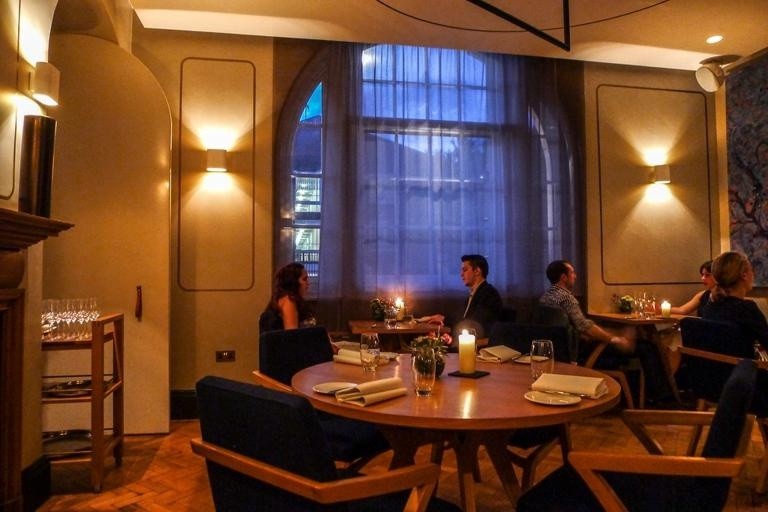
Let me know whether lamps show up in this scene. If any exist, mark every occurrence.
[693,63,746,94]
[24,61,62,108]
[647,164,671,186]
[203,148,233,174]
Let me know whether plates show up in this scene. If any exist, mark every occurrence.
[311,382,357,396]
[523,390,582,407]
[513,355,548,364]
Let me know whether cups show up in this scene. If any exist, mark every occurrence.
[632,293,655,320]
[407,307,413,324]
[360,332,380,373]
[530,341,555,379]
[367,309,376,328]
[410,347,436,396]
[383,308,396,330]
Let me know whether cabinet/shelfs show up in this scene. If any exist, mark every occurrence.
[39,309,127,494]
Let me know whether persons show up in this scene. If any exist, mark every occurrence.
[665,261,720,372]
[539,261,659,389]
[705,252,768,364]
[259,263,310,330]
[423,255,504,337]
